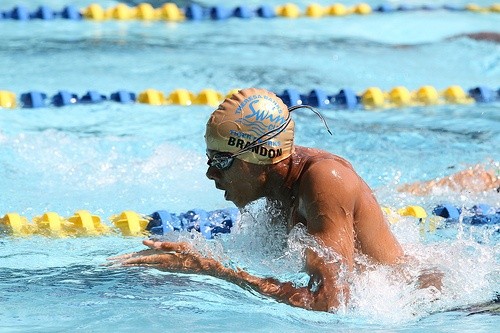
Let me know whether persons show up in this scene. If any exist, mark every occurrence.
[204,88,404,263]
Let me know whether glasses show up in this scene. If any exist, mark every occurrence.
[205,104,332,171]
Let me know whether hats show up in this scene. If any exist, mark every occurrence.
[205,87,295,164]
